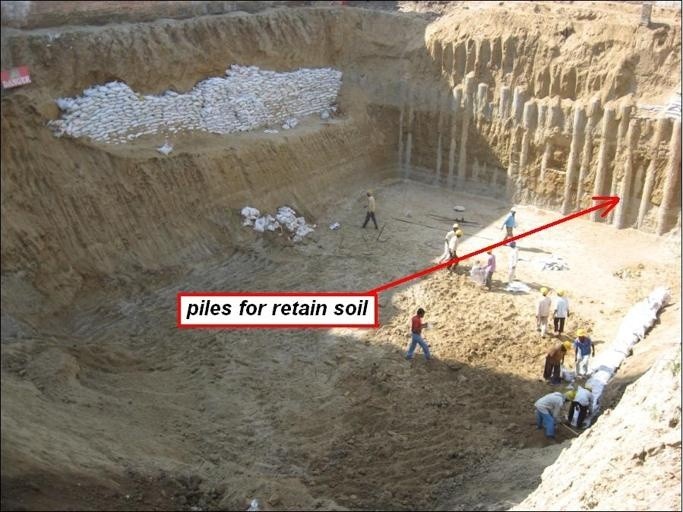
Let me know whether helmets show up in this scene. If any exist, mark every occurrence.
[563,341,572,352]
[511,206,517,212]
[584,385,592,391]
[539,287,549,295]
[575,329,586,337]
[453,223,463,236]
[565,390,576,400]
[557,288,564,295]
[486,248,492,253]
[366,189,374,195]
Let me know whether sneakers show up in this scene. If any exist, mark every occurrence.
[534,327,562,338]
[535,421,586,439]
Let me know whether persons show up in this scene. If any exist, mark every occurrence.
[572,328,595,380]
[534,287,552,338]
[498,207,518,248]
[476,248,496,291]
[532,390,574,440]
[549,288,570,336]
[542,340,571,386]
[436,223,458,264]
[445,230,463,271]
[504,240,517,284]
[469,259,482,298]
[403,308,435,361]
[360,189,378,230]
[563,384,594,430]
